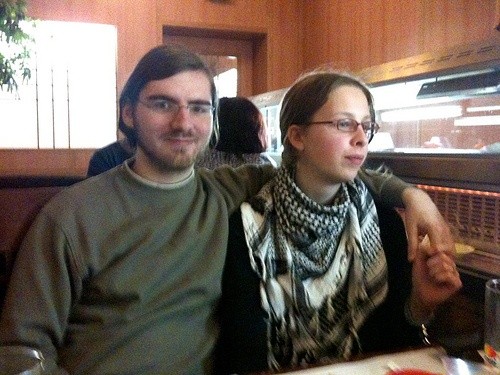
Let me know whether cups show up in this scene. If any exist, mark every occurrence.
[0,346,45,375]
[484,279,500,367]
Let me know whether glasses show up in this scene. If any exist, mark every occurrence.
[137,99,215,113]
[287,118,379,134]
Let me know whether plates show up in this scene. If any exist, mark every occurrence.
[454,243,475,258]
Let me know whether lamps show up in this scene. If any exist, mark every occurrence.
[416,70,500,99]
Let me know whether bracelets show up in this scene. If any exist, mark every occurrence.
[403,299,436,328]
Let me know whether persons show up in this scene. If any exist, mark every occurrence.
[223,67,463,375]
[194,96,276,167]
[86,125,134,178]
[0,43,459,375]
